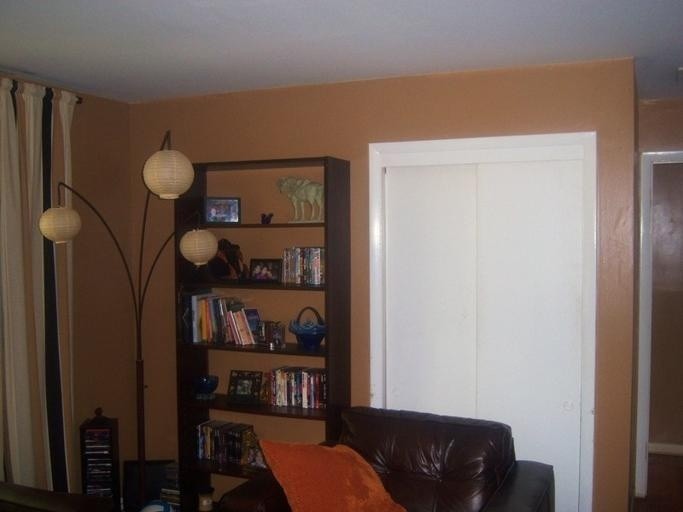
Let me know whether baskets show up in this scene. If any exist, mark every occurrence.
[289,307,325,347]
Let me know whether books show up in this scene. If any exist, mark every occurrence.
[269,366,327,410]
[180,289,259,346]
[281,245,324,285]
[192,419,254,465]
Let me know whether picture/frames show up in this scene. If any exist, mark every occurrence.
[228,369,263,400]
[203,195,242,224]
[249,258,283,283]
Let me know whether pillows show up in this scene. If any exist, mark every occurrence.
[257,440,406,512]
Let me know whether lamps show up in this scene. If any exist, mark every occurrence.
[40,129,218,459]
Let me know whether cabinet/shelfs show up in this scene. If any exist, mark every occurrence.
[173,155,352,484]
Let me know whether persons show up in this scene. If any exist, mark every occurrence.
[256,264,272,280]
[252,263,261,277]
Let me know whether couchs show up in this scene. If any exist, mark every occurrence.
[214,403,555,512]
